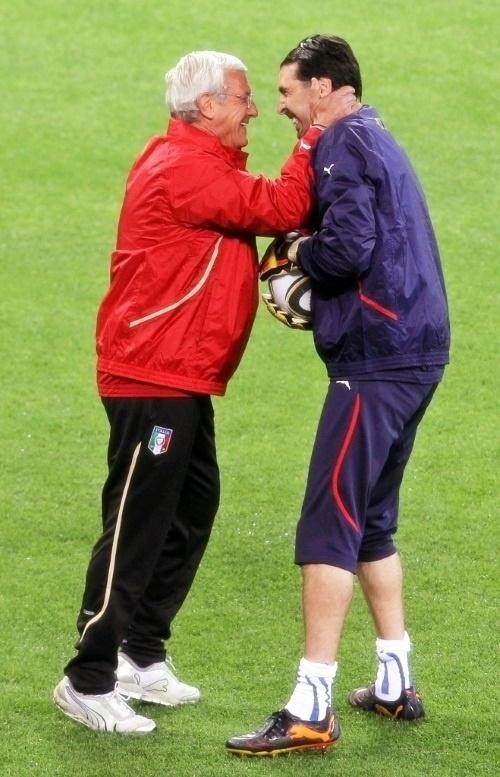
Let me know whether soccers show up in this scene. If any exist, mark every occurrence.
[268,265,314,320]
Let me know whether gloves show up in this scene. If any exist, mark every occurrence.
[257,229,312,281]
[262,292,316,331]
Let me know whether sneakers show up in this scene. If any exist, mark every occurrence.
[116,652,200,707]
[52,675,157,734]
[349,686,424,721]
[226,709,342,758]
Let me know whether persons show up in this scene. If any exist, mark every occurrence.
[222,29,456,756]
[48,48,364,739]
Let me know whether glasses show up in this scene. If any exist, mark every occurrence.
[217,91,255,109]
